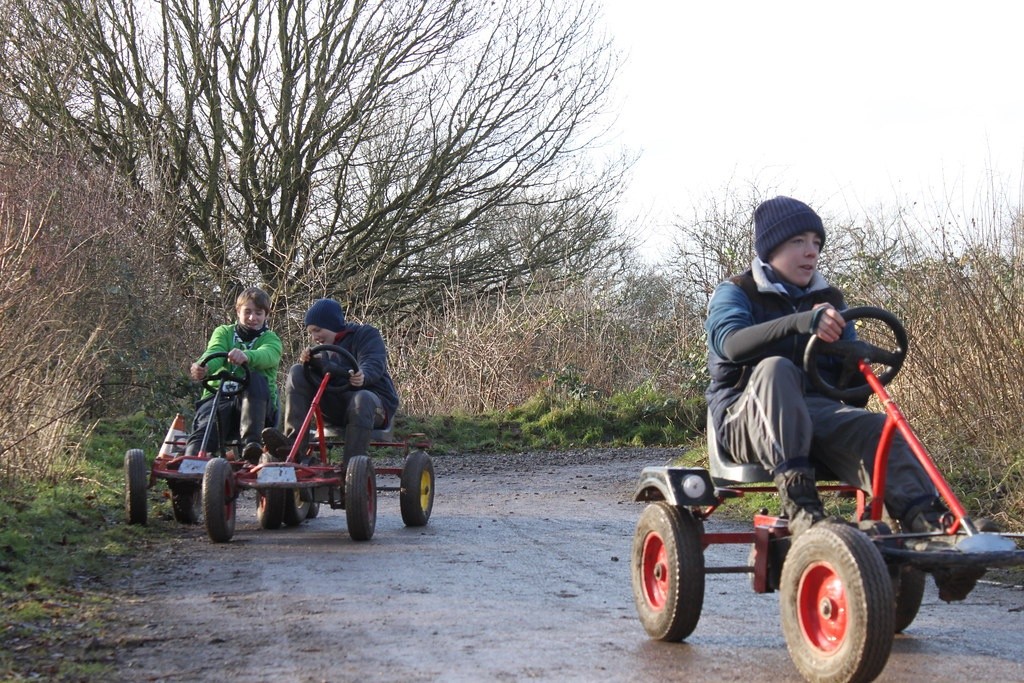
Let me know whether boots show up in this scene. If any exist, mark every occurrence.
[185,441,201,457]
[260,394,309,463]
[907,508,1004,603]
[772,456,848,549]
[335,426,372,485]
[240,396,263,465]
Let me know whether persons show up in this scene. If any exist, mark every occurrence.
[261,300,399,479]
[705,196,999,602]
[185,287,282,459]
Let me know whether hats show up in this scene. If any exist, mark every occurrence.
[304,299,345,334]
[754,196,826,263]
